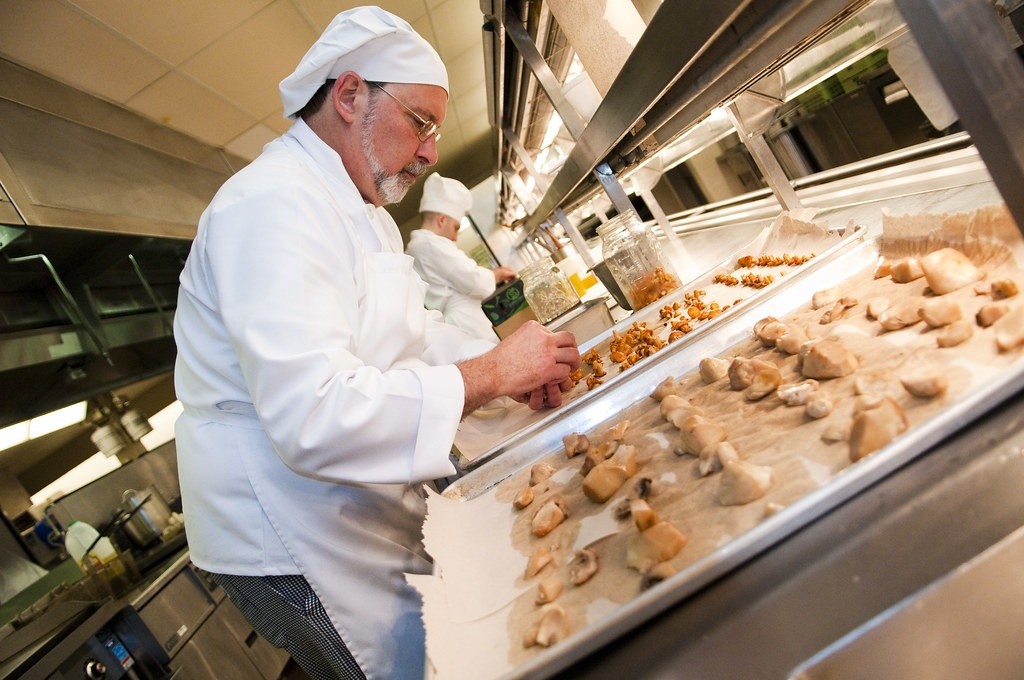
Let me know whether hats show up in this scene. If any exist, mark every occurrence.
[419,171,473,223]
[278,5,449,121]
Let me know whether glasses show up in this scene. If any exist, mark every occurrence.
[376,82,442,143]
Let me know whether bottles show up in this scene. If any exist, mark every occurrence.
[65,519,118,572]
[518,255,582,326]
[595,207,683,313]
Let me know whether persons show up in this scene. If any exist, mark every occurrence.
[405,172,516,344]
[172,4,579,680]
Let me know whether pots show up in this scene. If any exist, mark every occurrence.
[110,484,172,546]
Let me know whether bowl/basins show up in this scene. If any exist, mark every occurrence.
[586,242,653,311]
